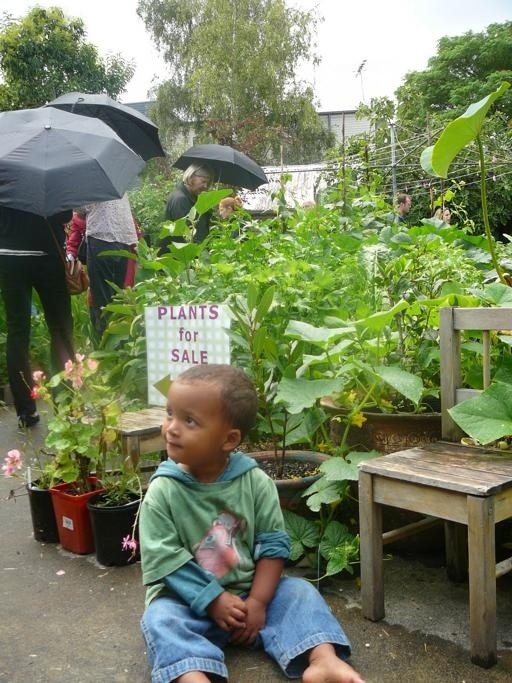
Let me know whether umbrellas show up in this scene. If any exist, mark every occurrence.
[0,106,148,221]
[41,90,169,160]
[171,142,268,198]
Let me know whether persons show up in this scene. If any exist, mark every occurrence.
[433,206,452,227]
[218,194,251,242]
[391,193,412,228]
[63,207,144,329]
[72,189,138,341]
[139,363,367,683]
[152,163,216,258]
[1,204,76,429]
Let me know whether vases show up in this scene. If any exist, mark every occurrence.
[87,488,152,574]
[46,476,113,559]
[25,472,66,552]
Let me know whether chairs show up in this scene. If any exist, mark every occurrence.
[345,297,512,674]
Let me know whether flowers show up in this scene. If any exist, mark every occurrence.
[30,351,120,494]
[0,355,62,487]
[91,423,144,562]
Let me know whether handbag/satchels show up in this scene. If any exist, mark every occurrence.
[65,253,90,295]
[78,240,87,265]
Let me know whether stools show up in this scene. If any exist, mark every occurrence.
[102,406,169,480]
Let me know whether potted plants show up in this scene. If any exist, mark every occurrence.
[341,249,492,468]
[226,287,351,528]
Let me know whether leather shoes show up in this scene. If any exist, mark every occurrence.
[17,413,40,428]
